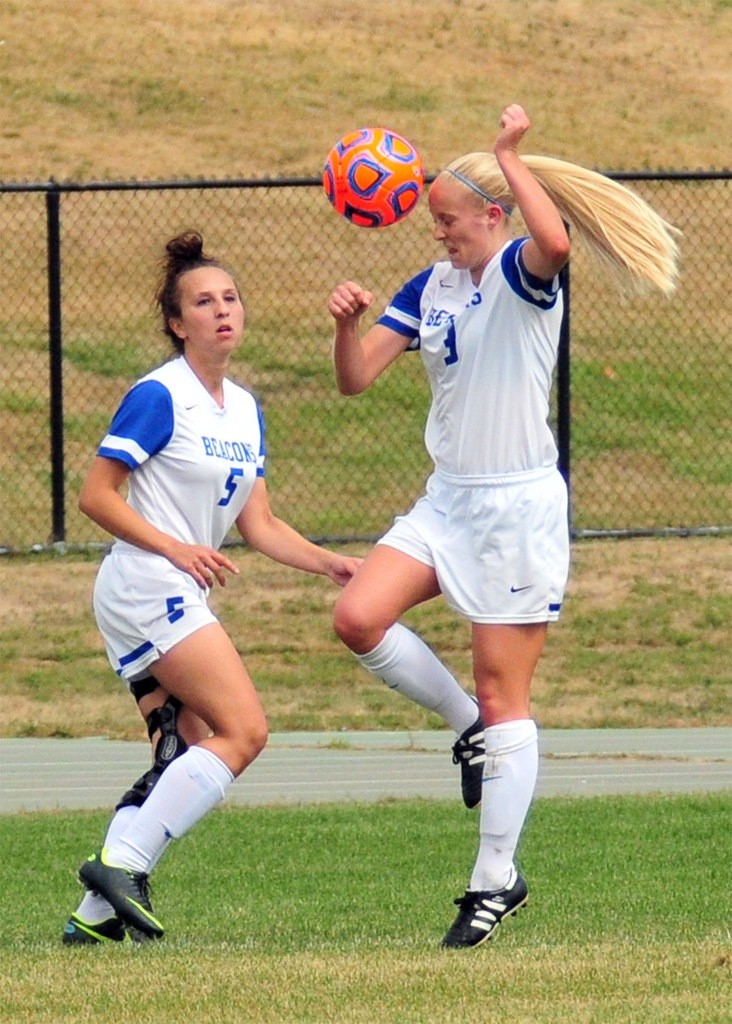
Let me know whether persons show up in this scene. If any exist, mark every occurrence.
[330,104,680,952]
[65,229,366,941]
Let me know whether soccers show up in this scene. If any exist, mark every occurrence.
[322,126,424,229]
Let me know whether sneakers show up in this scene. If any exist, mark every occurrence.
[439,860,530,948]
[78,847,165,940]
[451,695,486,809]
[62,912,144,949]
